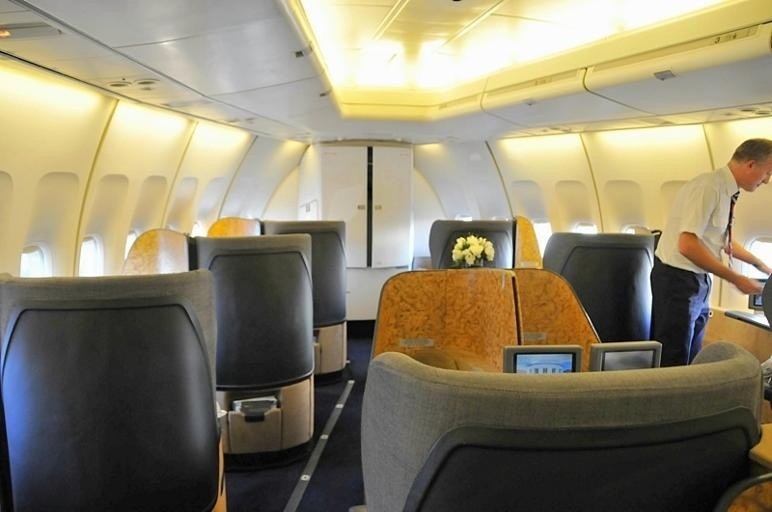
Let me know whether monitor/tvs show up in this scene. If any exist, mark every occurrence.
[749,279,766,309]
[588,340,662,373]
[503,345,582,374]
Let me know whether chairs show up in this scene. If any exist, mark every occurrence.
[410,213,772,342]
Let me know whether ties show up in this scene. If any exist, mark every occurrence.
[727,190,737,272]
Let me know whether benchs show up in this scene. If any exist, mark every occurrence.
[207,214,354,380]
[1,262,232,512]
[115,224,321,462]
[360,336,772,508]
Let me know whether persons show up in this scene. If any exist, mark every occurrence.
[651,139,772,368]
